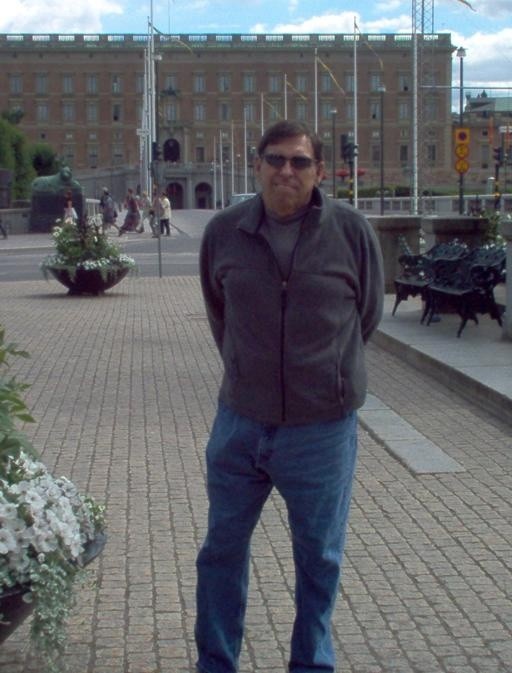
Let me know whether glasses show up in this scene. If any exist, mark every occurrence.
[265,154,312,169]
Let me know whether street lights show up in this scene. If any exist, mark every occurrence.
[152,53,162,144]
[454,45,469,215]
[327,109,340,200]
[205,145,256,209]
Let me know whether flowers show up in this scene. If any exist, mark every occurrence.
[0,321,108,673]
[38,208,136,275]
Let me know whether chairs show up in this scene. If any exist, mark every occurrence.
[422,245,509,340]
[387,240,471,326]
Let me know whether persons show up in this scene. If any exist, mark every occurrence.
[193,119,385,673]
[63,190,78,226]
[98,186,171,238]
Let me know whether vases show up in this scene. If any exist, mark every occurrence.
[0,525,105,645]
[46,263,130,300]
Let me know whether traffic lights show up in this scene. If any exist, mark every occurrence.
[491,144,505,169]
[344,139,360,164]
